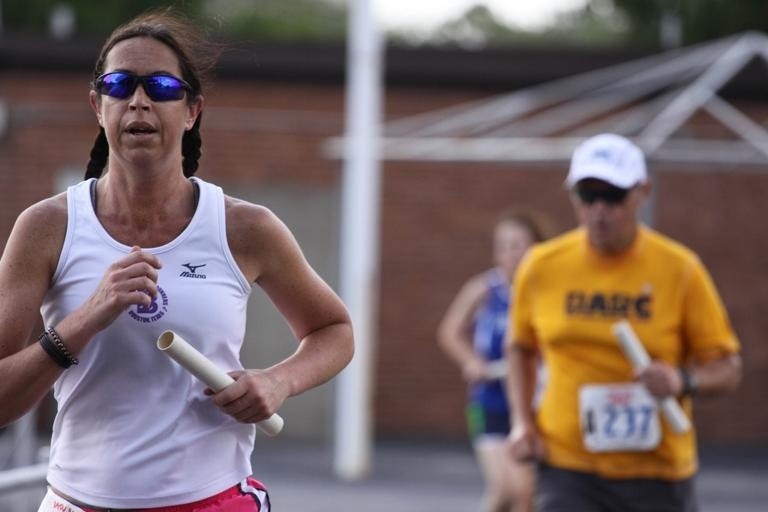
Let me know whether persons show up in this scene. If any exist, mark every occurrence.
[437,205,551,511]
[502,131,746,511]
[1,6,355,512]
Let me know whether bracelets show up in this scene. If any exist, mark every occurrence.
[678,367,701,396]
[36,327,80,370]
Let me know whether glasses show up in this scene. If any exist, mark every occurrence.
[93,70,196,103]
[573,182,640,204]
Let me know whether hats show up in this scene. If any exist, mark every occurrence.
[567,133,648,190]
